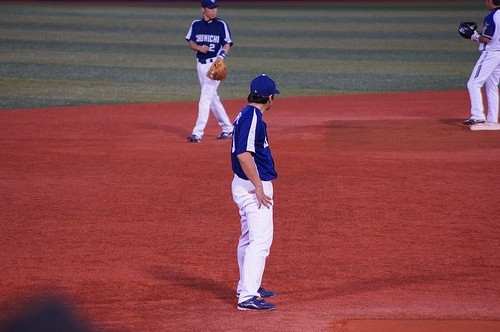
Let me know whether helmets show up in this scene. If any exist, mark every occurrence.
[457,21,477,38]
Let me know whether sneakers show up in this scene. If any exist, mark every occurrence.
[186,135,201,143]
[217,132,233,139]
[236,287,276,298]
[237,296,277,312]
[462,118,486,125]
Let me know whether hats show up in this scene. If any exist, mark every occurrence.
[249,74,280,96]
[201,0,218,8]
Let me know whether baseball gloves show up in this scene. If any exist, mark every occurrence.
[458,21,478,38]
[206,60,226,80]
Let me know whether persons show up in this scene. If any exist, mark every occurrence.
[185,0,233,143]
[462,0,500,125]
[231,74,281,311]
